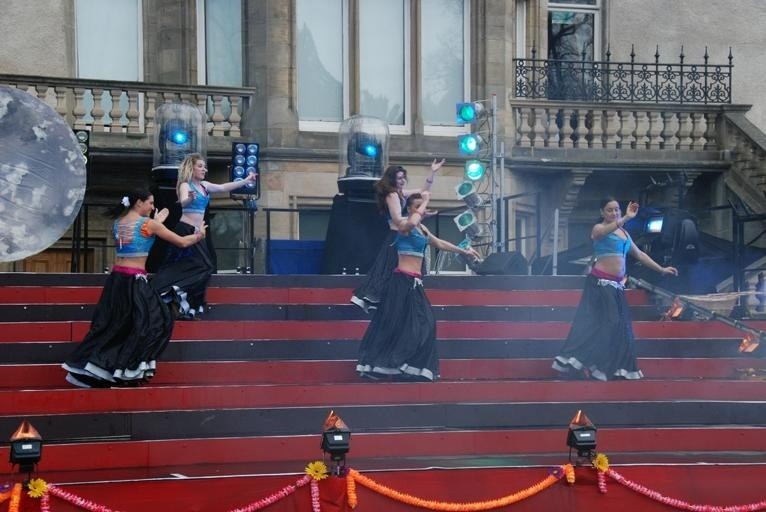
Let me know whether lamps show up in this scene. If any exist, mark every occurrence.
[321,430,351,470]
[230,139,261,273]
[566,426,596,463]
[9,439,42,480]
[347,132,383,175]
[452,99,489,263]
[159,117,197,164]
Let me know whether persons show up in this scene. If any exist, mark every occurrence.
[552,197,679,381]
[351,157,446,313]
[150,152,259,319]
[356,191,478,383]
[61,188,209,387]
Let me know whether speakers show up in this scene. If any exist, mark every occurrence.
[229,141,260,200]
[474,251,529,275]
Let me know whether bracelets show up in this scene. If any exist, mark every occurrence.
[426,178,434,183]
[414,211,422,218]
